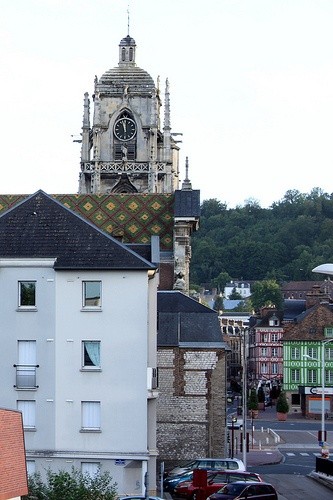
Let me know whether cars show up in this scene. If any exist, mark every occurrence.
[206,480,279,500]
[163,467,263,500]
[118,495,166,500]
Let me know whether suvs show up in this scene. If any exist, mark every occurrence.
[160,457,246,480]
[226,391,234,397]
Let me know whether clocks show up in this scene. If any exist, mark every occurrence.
[113,117,138,142]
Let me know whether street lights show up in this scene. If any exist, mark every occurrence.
[302,339,333,458]
[244,326,248,440]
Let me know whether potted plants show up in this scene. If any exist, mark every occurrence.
[275,393,289,420]
[246,390,259,419]
[257,387,265,409]
[269,386,278,406]
